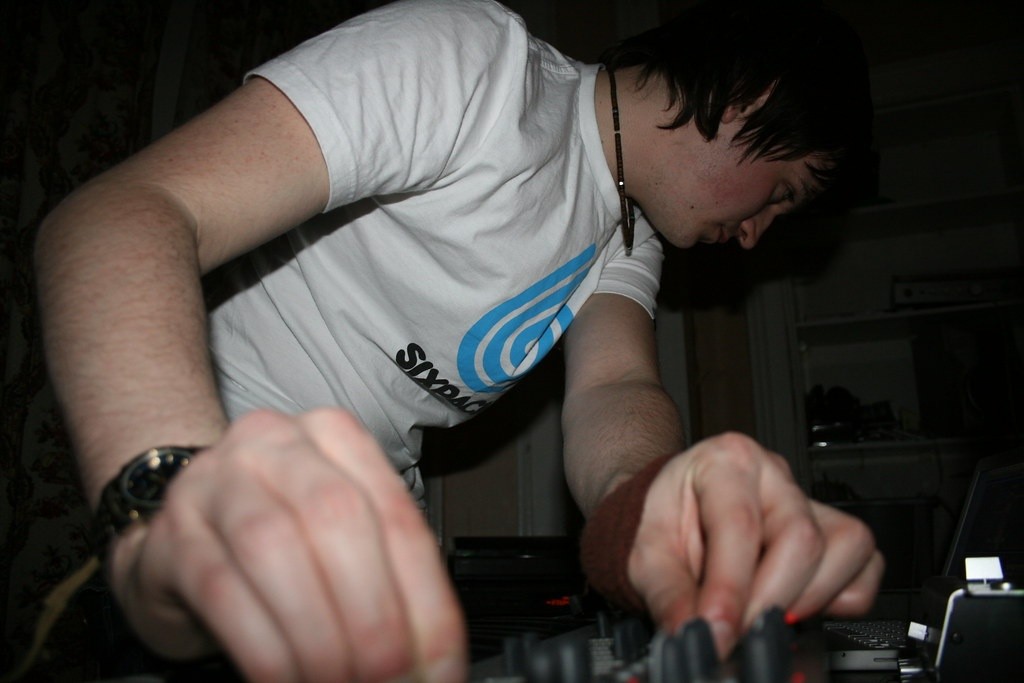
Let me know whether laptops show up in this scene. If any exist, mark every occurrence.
[825,459,1024,671]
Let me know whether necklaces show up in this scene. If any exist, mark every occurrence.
[605,64,635,257]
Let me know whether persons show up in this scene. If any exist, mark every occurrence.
[32,0,887,683]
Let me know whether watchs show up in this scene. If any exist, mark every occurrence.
[84,445,210,561]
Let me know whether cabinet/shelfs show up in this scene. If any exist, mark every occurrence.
[779,83,1024,510]
